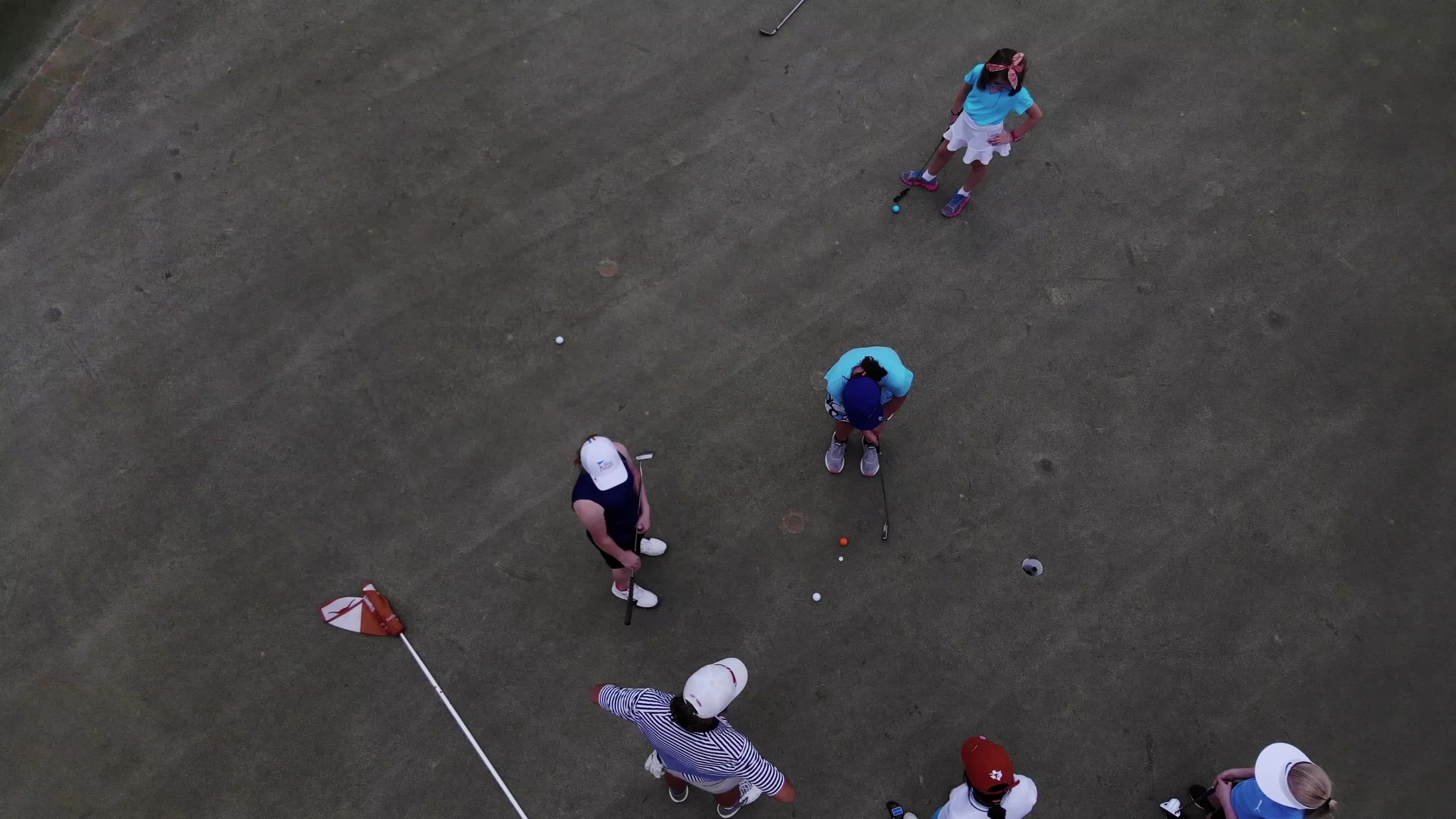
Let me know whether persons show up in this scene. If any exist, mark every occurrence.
[903,736,1037,819]
[591,656,795,819]
[1190,741,1338,819]
[572,435,667,609]
[824,346,914,477]
[900,49,1044,218]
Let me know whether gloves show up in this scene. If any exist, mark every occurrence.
[643,750,665,778]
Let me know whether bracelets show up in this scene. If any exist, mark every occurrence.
[951,108,962,116]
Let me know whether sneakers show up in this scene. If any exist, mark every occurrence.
[825,431,848,474]
[638,538,667,556]
[941,193,971,217]
[901,169,938,190]
[611,581,657,607]
[717,779,763,818]
[669,785,688,802]
[860,436,880,477]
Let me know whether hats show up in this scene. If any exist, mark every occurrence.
[1254,742,1323,810]
[961,736,1020,796]
[580,436,627,492]
[842,377,883,431]
[683,656,748,719]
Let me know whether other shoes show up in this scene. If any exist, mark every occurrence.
[904,813,918,819]
[1190,784,1215,810]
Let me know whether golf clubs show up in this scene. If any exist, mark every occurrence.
[875,432,890,540]
[892,122,957,203]
[759,0,804,35]
[1160,780,1230,817]
[623,452,656,628]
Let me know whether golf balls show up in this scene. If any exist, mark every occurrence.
[892,205,900,213]
[812,592,822,601]
[839,537,847,546]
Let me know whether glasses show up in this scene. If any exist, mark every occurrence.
[991,81,1012,89]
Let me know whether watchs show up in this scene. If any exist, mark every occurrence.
[1010,129,1022,142]
